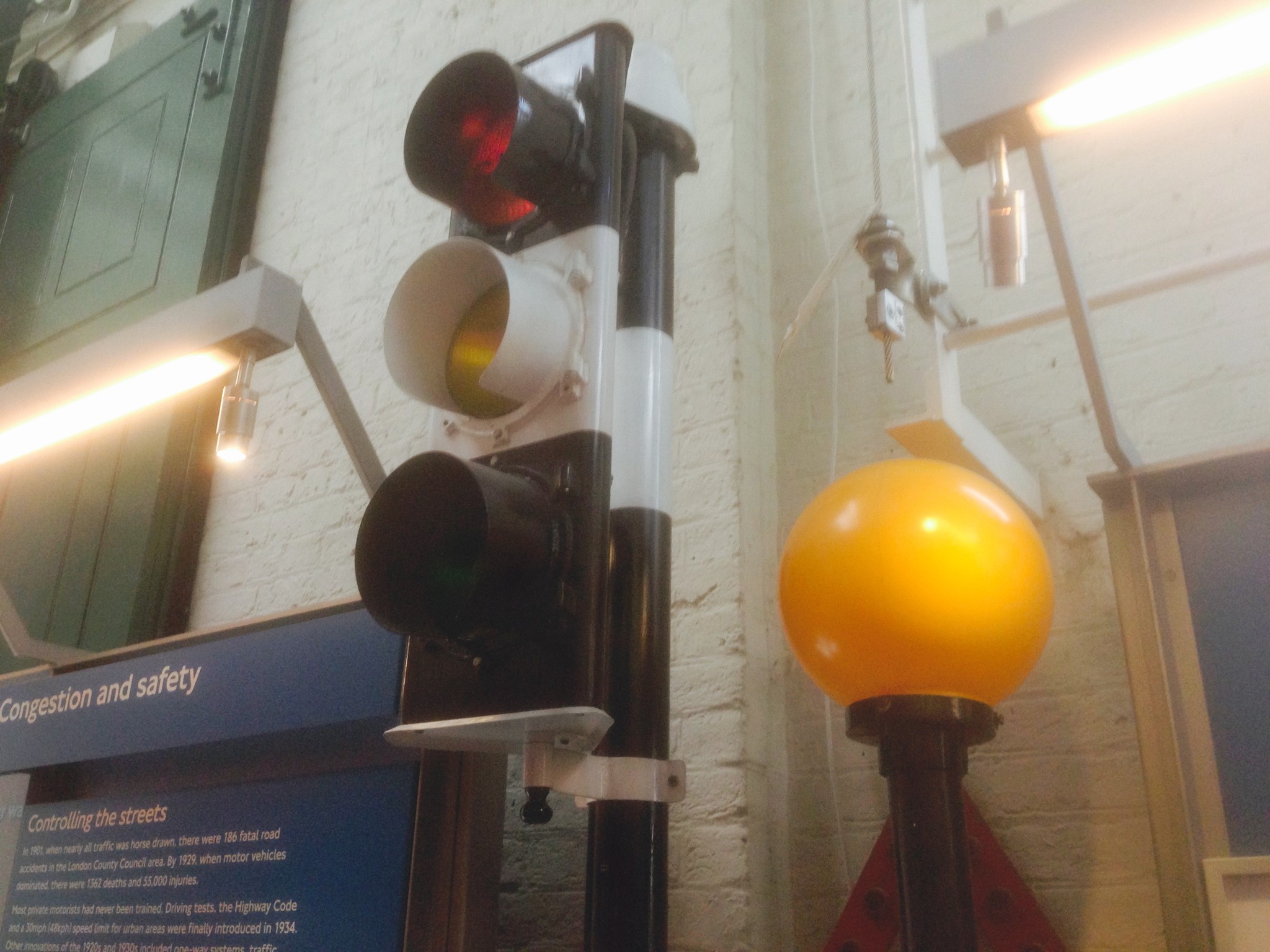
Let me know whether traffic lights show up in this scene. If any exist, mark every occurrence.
[351,18,637,752]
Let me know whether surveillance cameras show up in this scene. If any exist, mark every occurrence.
[989,186,1030,286]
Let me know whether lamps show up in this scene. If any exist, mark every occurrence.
[0,269,393,665]
[930,0,1270,476]
[967,128,1036,295]
[212,345,271,467]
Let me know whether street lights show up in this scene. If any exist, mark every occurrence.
[774,455,1057,951]
